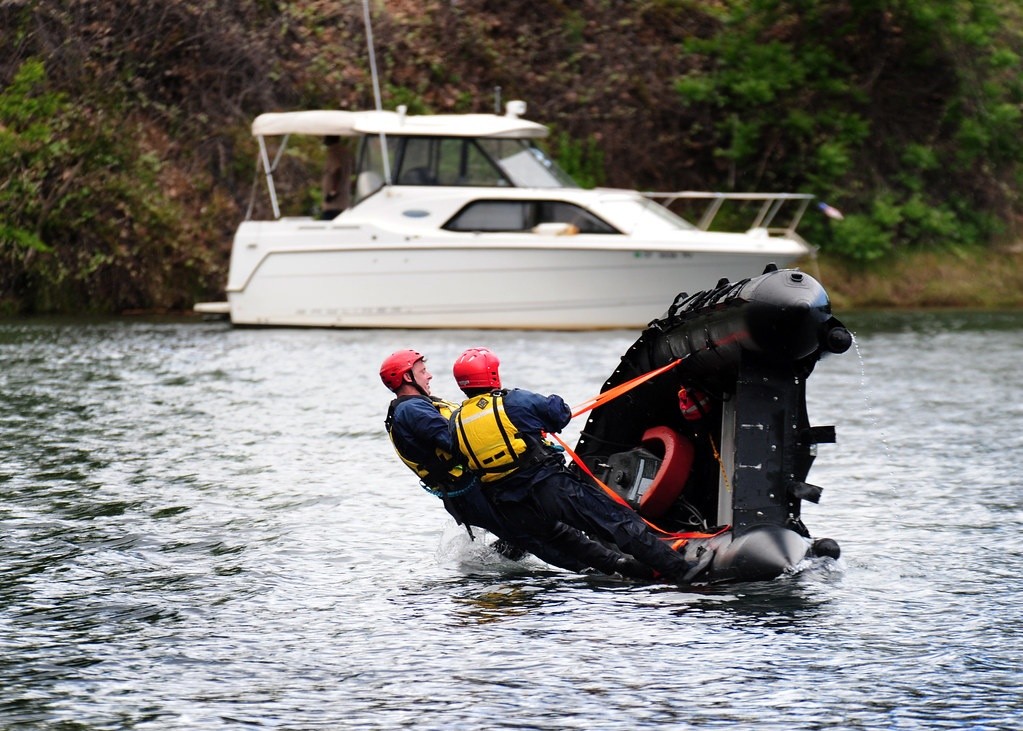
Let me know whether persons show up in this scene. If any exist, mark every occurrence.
[448,348,714,584]
[380,348,657,582]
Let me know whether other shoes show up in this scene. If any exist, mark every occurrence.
[683,548,715,582]
[615,556,663,581]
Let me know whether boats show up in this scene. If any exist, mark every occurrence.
[574,268,852,582]
[193,105,830,330]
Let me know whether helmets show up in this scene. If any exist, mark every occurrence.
[453,347,501,389]
[379,349,424,392]
